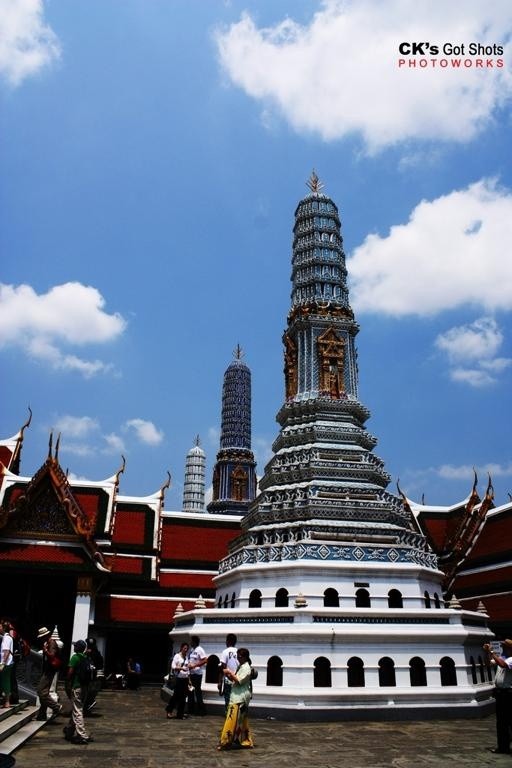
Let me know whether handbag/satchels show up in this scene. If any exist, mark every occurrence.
[251,668,258,679]
[13,637,30,662]
[168,676,177,689]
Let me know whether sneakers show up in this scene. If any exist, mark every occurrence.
[52,703,62,719]
[87,699,96,711]
[31,714,47,721]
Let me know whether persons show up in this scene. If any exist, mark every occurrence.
[127,658,140,689]
[164,641,187,720]
[215,634,240,718]
[188,636,209,715]
[216,648,254,750]
[482,639,512,755]
[0,615,103,744]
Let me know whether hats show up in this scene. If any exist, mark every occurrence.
[37,627,51,638]
